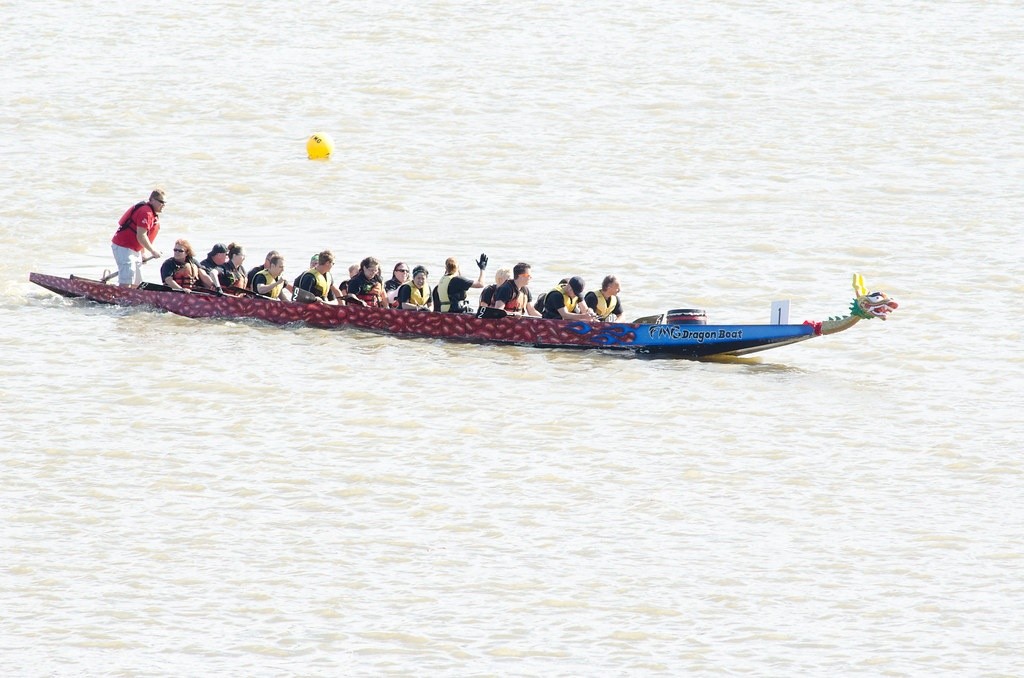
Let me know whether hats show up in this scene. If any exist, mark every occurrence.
[569,276,585,297]
[207,243,227,255]
[413,266,428,277]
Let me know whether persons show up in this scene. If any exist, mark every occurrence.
[161,238,624,323]
[112,189,167,288]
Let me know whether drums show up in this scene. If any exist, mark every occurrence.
[666,308,706,325]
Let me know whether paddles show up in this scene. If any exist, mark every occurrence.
[345,297,370,307]
[227,284,277,301]
[477,305,544,318]
[197,286,241,298]
[138,282,212,295]
[101,251,163,282]
[291,286,331,305]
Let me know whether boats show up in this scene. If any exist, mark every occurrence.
[30,271,898,356]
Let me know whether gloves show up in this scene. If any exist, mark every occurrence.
[476,253,488,270]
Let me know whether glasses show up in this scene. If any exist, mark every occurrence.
[331,262,335,265]
[154,197,166,204]
[395,269,409,273]
[518,273,533,279]
[173,248,185,252]
